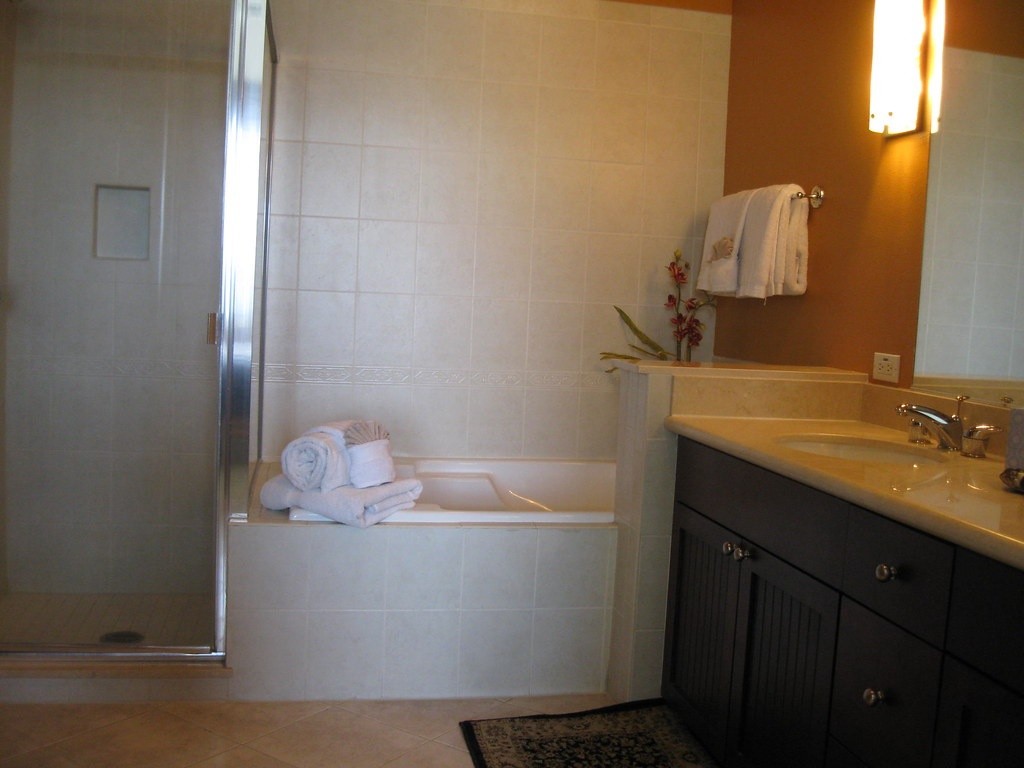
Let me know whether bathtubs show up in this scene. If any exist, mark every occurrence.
[227,458,620,703]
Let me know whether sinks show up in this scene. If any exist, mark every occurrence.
[771,433,950,467]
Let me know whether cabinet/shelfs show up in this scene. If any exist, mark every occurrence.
[660,434,1024,768]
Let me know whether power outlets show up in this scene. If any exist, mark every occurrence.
[872,352,900,383]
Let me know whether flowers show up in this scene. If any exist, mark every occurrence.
[598,251,718,373]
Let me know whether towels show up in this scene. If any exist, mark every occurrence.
[696,183,809,299]
[258,419,423,529]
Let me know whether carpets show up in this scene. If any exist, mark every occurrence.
[459,696,719,768]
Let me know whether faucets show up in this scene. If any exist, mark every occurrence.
[894,402,963,451]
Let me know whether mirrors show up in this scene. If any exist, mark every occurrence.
[909,1,1024,413]
